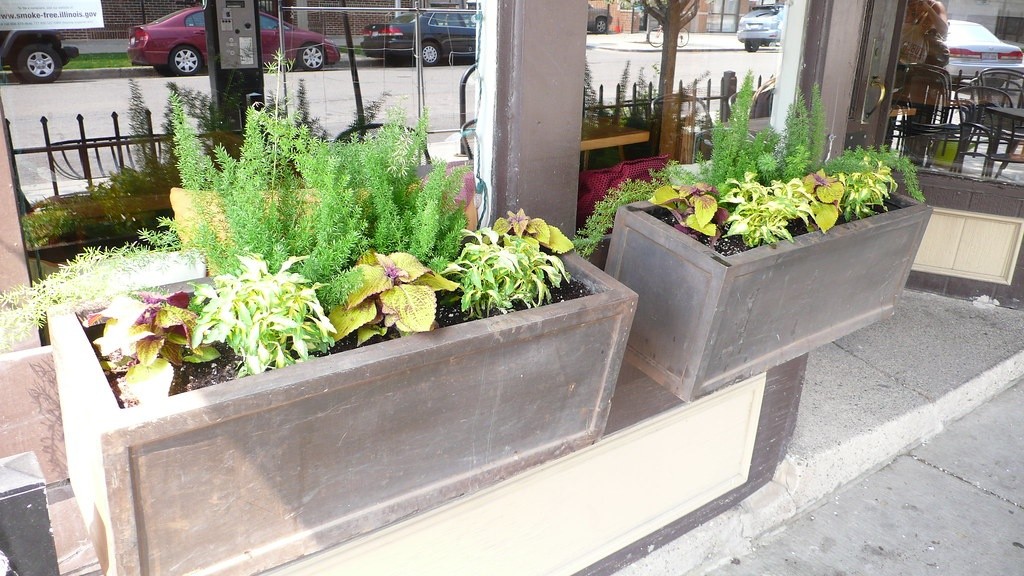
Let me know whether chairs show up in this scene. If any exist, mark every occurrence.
[651,89,775,164]
[333,123,431,165]
[885,64,1024,181]
[461,119,477,163]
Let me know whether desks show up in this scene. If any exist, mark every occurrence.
[580,117,649,170]
[986,106,1024,181]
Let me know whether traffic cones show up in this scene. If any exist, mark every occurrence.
[613,16,620,33]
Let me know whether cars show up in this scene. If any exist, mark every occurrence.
[941,20,1024,92]
[587,3,612,33]
[361,9,477,67]
[126,4,341,76]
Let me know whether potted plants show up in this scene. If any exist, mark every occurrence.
[0,48,640,576]
[572,69,933,406]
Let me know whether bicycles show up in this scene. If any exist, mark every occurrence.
[647,25,689,48]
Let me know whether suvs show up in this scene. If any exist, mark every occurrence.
[736,2,788,52]
